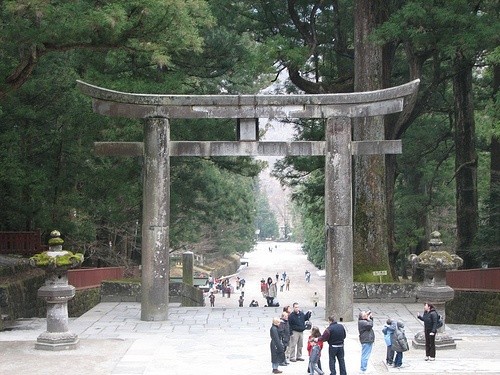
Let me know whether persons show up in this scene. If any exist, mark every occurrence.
[209,292,215,307]
[269,302,312,373]
[208,274,212,287]
[314,316,347,375]
[382,319,396,366]
[260,278,265,298]
[285,277,290,291]
[417,303,438,361]
[391,322,409,368]
[358,311,375,374]
[282,272,286,282]
[265,277,277,307]
[304,270,311,284]
[238,295,244,307]
[249,300,259,307]
[275,273,279,282]
[235,277,245,290]
[312,292,320,307]
[307,326,325,375]
[221,284,231,299]
[280,278,284,291]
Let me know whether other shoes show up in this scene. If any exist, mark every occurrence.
[290,359,296,362]
[430,357,435,360]
[425,357,429,360]
[273,370,282,373]
[280,363,286,366]
[296,358,303,361]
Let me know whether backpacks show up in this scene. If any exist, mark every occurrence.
[428,310,443,329]
